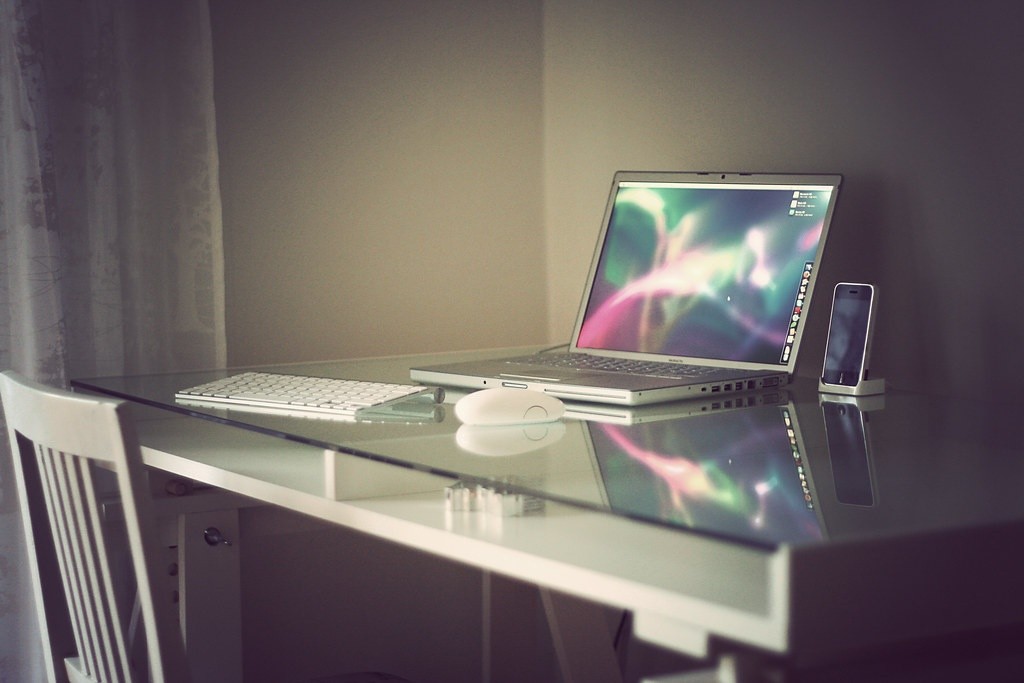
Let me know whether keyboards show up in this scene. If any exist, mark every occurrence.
[175,372,427,415]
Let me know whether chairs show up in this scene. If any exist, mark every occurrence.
[0,370,187,683]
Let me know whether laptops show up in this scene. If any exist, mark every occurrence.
[412,171,844,406]
[565,389,829,552]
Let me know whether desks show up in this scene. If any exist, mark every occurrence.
[43,343,1024,683]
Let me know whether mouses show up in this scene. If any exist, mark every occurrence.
[455,386,566,425]
[455,421,566,457]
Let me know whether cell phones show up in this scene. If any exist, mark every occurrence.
[822,402,880,511]
[822,283,878,387]
